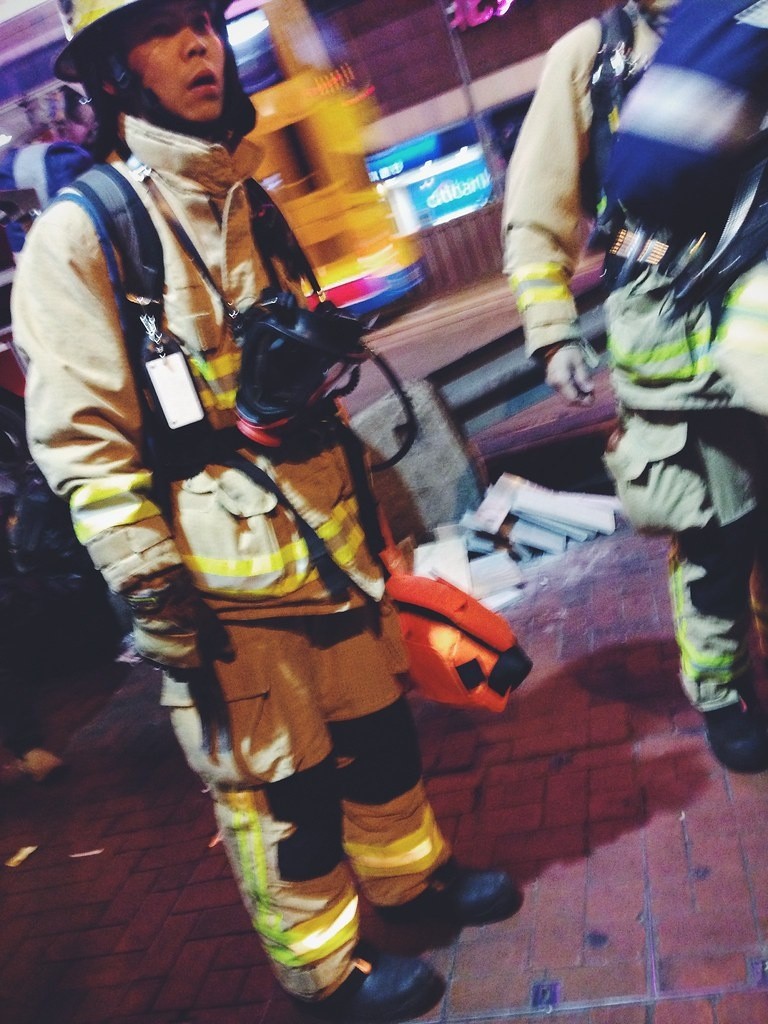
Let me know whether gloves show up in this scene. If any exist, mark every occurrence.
[117,577,238,678]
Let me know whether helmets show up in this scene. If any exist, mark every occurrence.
[53,0,234,84]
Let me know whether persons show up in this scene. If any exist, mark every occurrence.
[496,0,768,772]
[0,0,521,1024]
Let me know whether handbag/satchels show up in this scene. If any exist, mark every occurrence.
[380,572,535,710]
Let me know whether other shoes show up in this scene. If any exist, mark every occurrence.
[293,946,436,1023]
[377,867,515,923]
[701,694,768,774]
